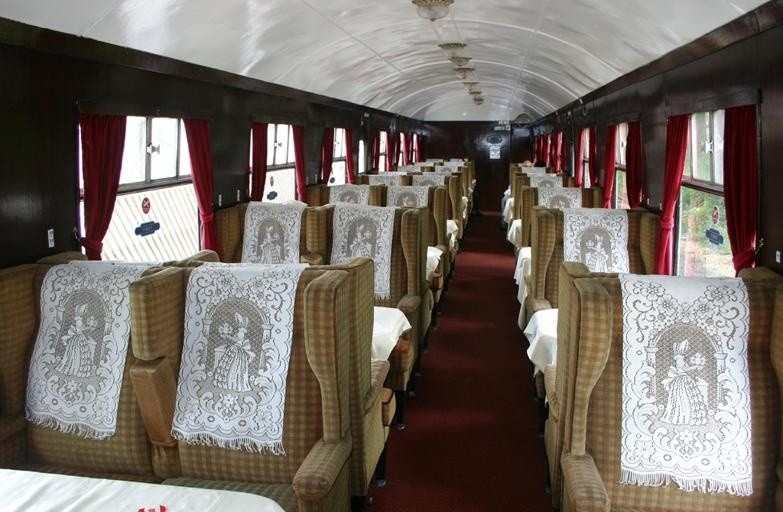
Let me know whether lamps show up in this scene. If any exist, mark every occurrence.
[411,1,485,106]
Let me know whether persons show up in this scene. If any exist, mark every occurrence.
[499,155,535,219]
